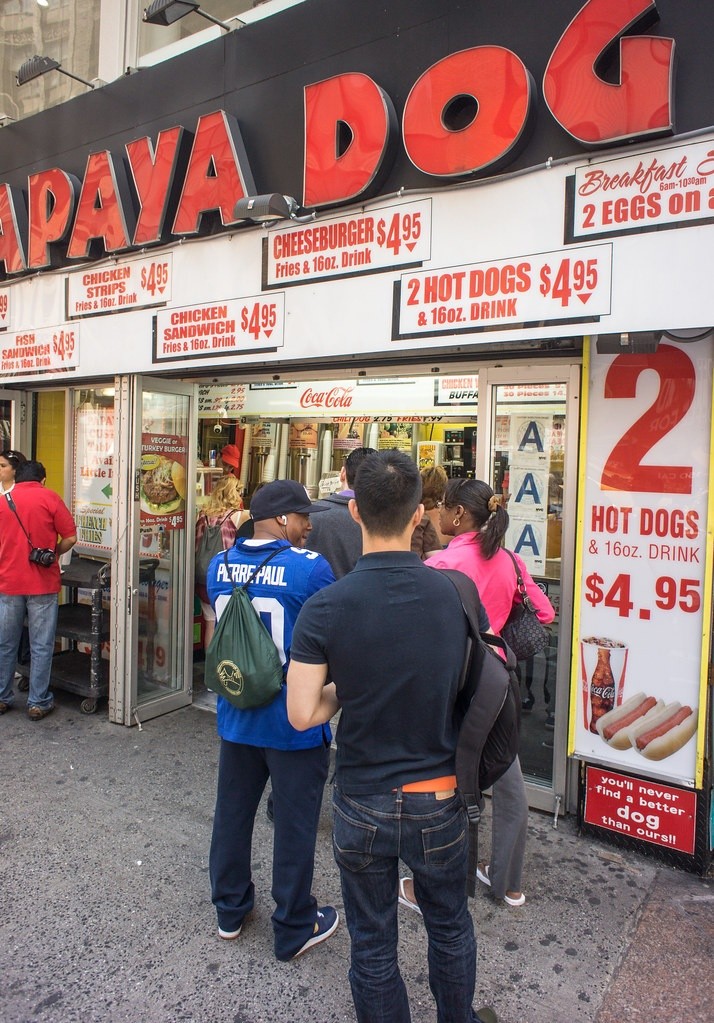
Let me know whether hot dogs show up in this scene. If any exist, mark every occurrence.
[596,691,665,750]
[628,700,698,761]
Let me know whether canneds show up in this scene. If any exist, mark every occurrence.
[209,450,216,468]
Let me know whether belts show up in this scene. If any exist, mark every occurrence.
[392,776,458,792]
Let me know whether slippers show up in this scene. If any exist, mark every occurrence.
[476,861,525,906]
[399,877,424,916]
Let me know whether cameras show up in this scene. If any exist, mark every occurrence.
[29,548,56,567]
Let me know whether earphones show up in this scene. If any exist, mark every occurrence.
[281,515,288,526]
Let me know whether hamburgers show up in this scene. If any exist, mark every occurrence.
[139,456,186,513]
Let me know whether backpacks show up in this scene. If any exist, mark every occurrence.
[195,509,234,587]
[441,568,522,825]
[205,546,283,711]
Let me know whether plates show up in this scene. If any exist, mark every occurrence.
[140,454,184,515]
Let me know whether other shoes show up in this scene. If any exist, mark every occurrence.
[522,698,533,714]
[0,700,8,715]
[546,712,555,729]
[476,1007,497,1023]
[29,702,54,720]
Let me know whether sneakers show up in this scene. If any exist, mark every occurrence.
[218,920,242,939]
[291,906,339,959]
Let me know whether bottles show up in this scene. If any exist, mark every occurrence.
[589,648,615,735]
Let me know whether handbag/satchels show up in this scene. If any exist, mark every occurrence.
[501,548,548,660]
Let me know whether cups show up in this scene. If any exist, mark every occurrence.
[278,423,289,481]
[368,423,378,450]
[321,431,331,476]
[579,641,627,734]
[262,454,275,484]
[238,423,251,488]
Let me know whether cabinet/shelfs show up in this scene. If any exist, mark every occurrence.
[15,551,160,716]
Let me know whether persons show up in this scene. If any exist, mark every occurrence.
[398,478,555,916]
[0,449,27,678]
[0,461,77,721]
[206,480,339,962]
[286,448,499,1023]
[195,444,449,822]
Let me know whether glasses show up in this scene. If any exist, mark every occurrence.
[5,450,20,463]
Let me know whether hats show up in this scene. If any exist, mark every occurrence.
[221,444,240,468]
[250,480,331,522]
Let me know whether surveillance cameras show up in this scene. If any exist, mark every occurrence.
[214,424,222,434]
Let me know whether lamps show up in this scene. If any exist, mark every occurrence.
[142,0,246,37]
[235,189,290,224]
[596,330,664,354]
[14,55,109,91]
